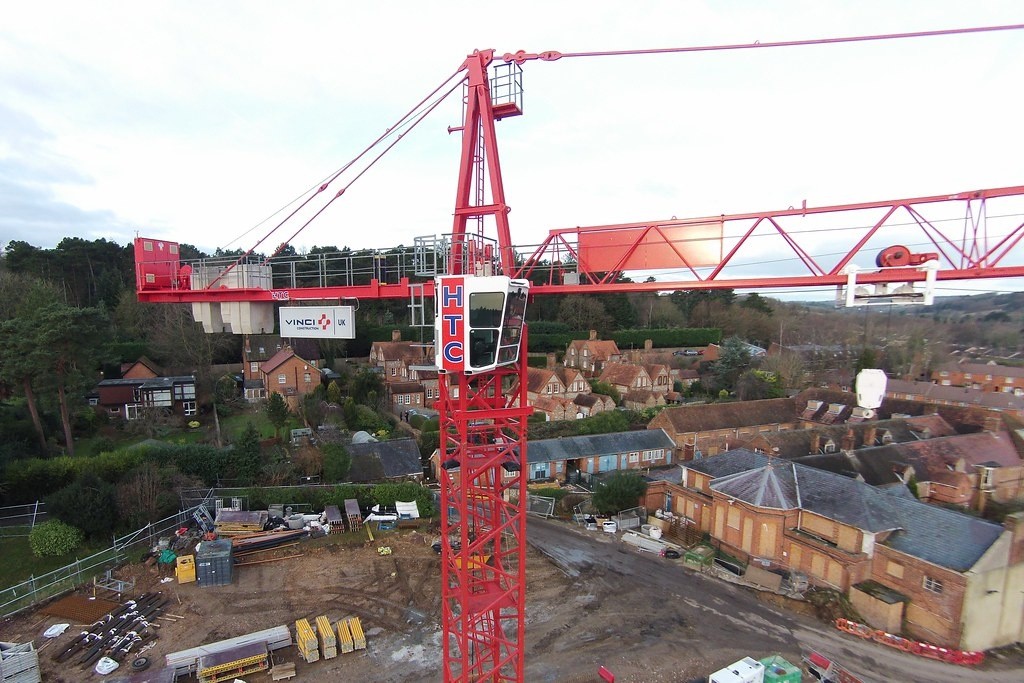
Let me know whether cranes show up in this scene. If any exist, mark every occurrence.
[133,22,1023,683]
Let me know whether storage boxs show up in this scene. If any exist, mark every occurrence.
[197,540,234,588]
[176,554,195,585]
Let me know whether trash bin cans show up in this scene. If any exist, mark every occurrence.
[175,555,196,584]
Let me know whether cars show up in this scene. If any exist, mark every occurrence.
[698,349,705,355]
[673,350,684,356]
[684,349,699,355]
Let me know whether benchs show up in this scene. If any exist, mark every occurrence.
[399,520,420,529]
